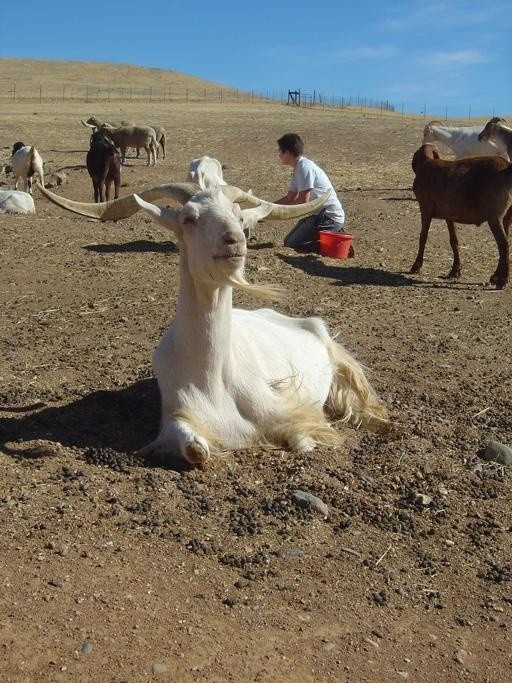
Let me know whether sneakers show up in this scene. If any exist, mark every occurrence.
[341,227,354,258]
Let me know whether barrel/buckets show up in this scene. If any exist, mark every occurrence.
[318,229,355,259]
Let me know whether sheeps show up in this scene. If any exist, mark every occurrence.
[187,155,228,191]
[34,156,395,464]
[409,117,512,289]
[81,116,167,202]
[12,141,44,194]
[0,190,37,213]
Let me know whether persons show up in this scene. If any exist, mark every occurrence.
[271,133,356,257]
